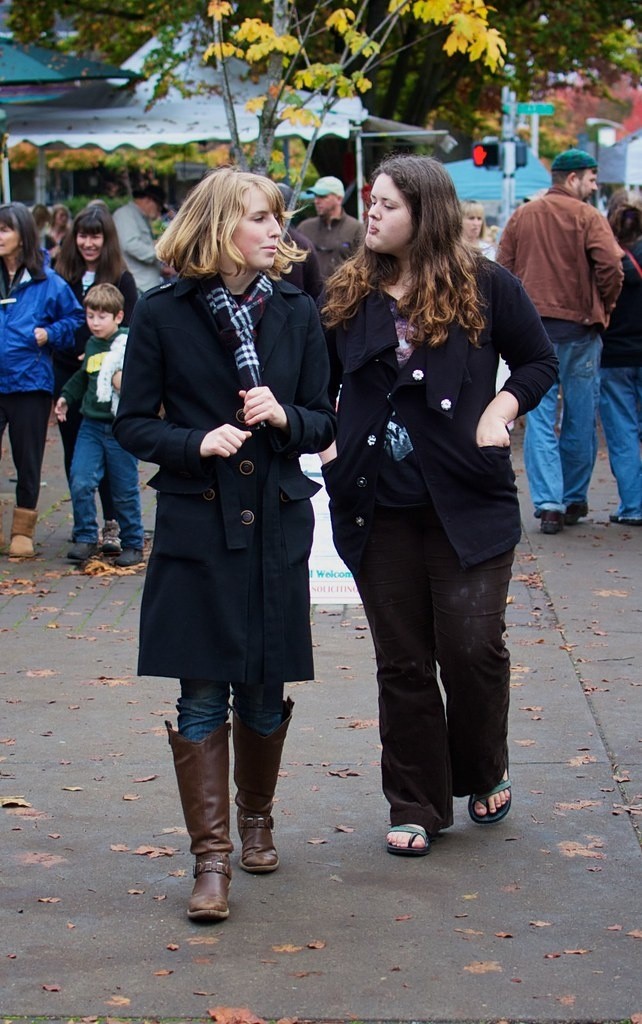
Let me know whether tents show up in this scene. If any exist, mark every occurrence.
[6,18,642,222]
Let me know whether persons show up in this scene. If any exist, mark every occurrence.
[0,149,642,567]
[111,168,339,919]
[312,156,560,857]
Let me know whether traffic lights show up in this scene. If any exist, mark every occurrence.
[472,142,502,167]
[516,143,527,168]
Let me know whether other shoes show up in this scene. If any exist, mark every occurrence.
[563,501,588,526]
[67,542,98,559]
[100,519,122,553]
[114,548,145,566]
[609,513,642,527]
[541,510,563,534]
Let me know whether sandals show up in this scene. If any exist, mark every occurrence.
[467,766,512,824]
[385,822,431,857]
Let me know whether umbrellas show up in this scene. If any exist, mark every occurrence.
[1,39,148,205]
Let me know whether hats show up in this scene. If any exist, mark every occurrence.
[307,176,345,199]
[132,185,170,216]
[551,148,598,170]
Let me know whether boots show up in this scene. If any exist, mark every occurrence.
[9,507,38,557]
[165,719,235,918]
[232,697,295,872]
